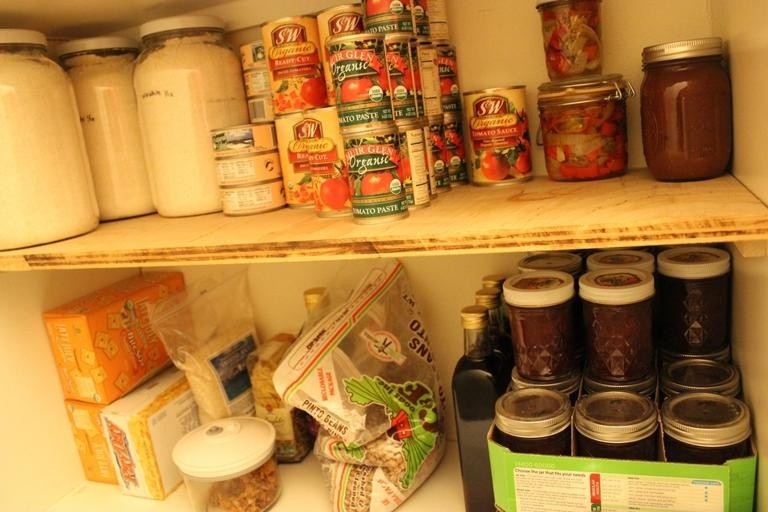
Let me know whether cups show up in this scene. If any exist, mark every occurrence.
[171,414,285,512]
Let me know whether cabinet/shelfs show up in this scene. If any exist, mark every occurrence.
[0,0,768,512]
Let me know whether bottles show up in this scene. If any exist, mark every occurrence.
[536,71,630,183]
[495,244,752,465]
[57,35,158,224]
[452,274,513,512]
[131,14,251,216]
[301,288,337,443]
[536,0,602,84]
[0,27,100,251]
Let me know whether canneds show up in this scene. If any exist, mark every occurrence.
[496,247,754,466]
[538,0,636,181]
[1,0,534,250]
